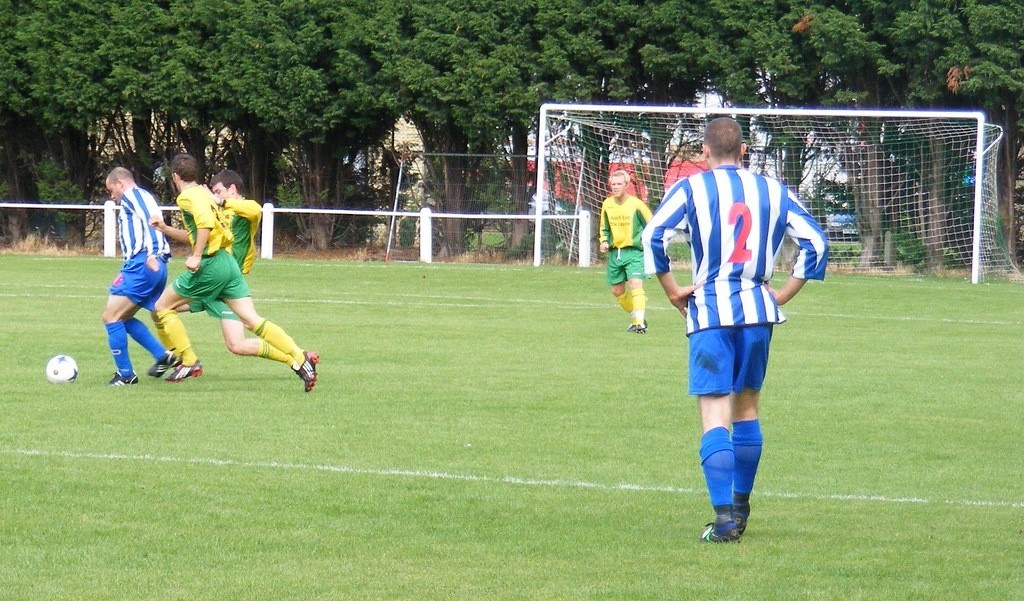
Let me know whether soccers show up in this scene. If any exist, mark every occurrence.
[45,354,79,384]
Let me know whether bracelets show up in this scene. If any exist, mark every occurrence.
[216,197,227,208]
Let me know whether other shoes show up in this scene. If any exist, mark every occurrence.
[701,520,740,543]
[628,320,648,335]
[733,503,750,533]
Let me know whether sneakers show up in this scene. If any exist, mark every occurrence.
[148,350,181,379]
[165,359,203,383]
[108,371,139,386]
[292,351,319,392]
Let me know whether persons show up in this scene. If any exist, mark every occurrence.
[150,171,296,378]
[599,170,653,334]
[147,153,319,392]
[102,166,182,387]
[641,118,829,543]
